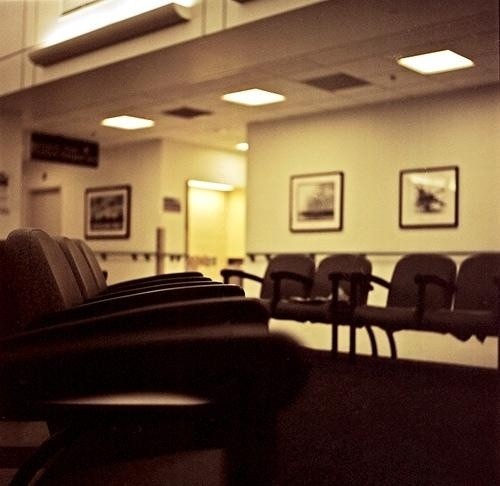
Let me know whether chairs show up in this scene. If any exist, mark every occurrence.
[220,252,500,371]
[0,227,309,486]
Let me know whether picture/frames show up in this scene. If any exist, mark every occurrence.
[288,170,344,233]
[82,184,130,239]
[398,165,459,229]
